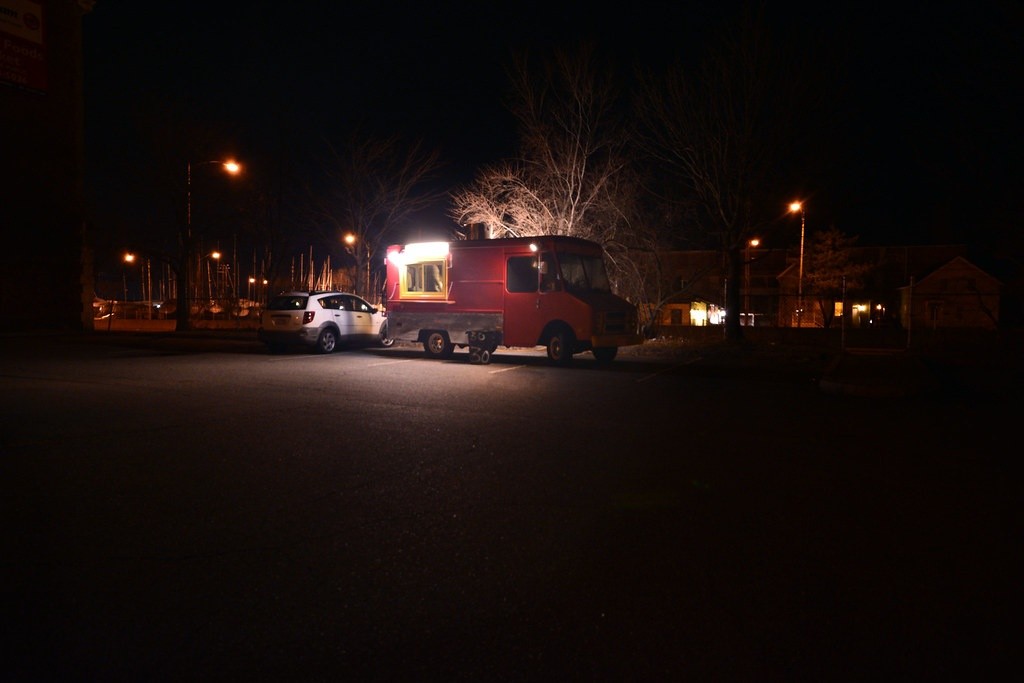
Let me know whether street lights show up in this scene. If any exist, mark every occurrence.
[200,252,221,304]
[790,202,804,330]
[124,255,134,318]
[344,232,370,301]
[186,159,240,242]
[744,238,759,327]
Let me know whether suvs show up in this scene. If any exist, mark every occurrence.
[254,291,395,353]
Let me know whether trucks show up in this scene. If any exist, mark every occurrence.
[384,234,648,369]
